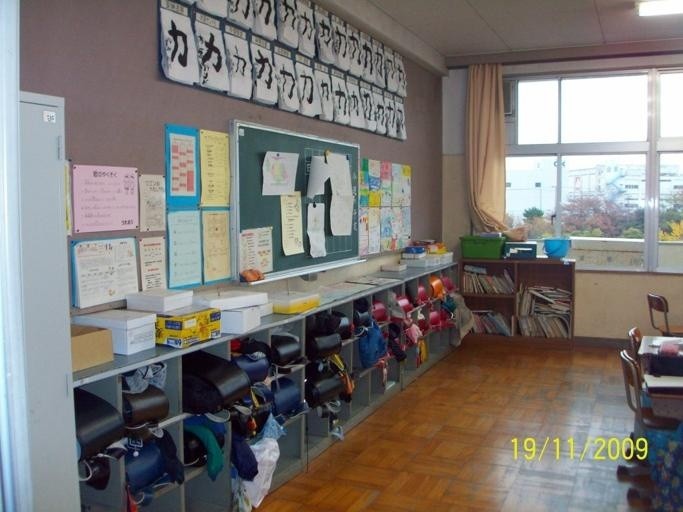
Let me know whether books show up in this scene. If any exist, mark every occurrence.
[460,263,515,294]
[517,279,571,340]
[470,310,510,337]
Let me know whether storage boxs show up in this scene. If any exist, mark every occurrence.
[125,289,194,313]
[643,382,682,421]
[72,324,113,374]
[220,305,261,334]
[74,309,157,355]
[459,236,505,259]
[505,241,537,260]
[155,305,221,349]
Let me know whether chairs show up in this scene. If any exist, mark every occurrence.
[628,327,646,391]
[647,293,683,336]
[620,349,678,464]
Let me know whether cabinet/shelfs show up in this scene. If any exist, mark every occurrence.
[363,261,460,390]
[73,276,404,511]
[454,253,582,344]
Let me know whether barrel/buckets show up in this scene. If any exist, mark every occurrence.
[543,240,571,258]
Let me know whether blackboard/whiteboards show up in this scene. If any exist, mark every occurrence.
[228,117,367,285]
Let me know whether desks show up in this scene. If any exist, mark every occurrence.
[618,336,683,507]
